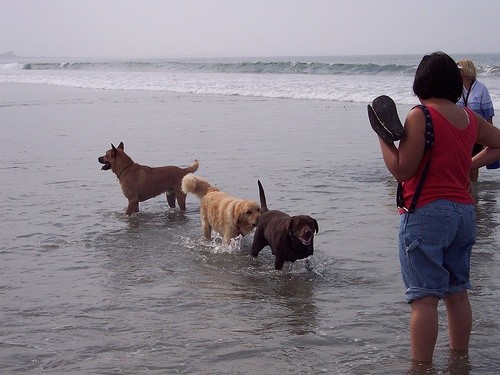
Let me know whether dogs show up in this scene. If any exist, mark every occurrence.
[98,142,199,215]
[182,172,260,246]
[251,178,319,271]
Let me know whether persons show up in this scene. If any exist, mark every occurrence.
[378,51,500,362]
[453,60,496,181]
